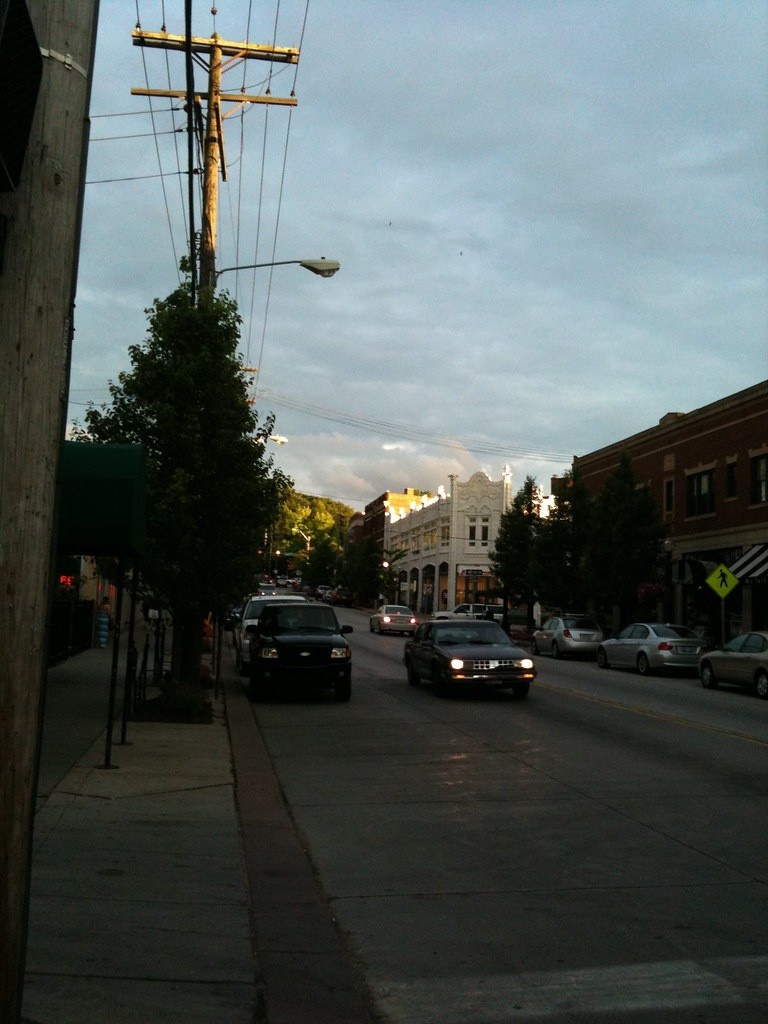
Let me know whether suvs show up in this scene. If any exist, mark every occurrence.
[246,603,355,703]
[227,594,312,676]
[314,585,353,607]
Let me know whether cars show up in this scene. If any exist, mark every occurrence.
[275,576,300,589]
[597,623,707,675]
[404,621,537,702]
[532,615,605,660]
[697,632,768,699]
[369,605,417,634]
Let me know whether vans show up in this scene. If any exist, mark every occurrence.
[256,583,276,596]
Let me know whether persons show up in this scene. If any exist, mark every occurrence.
[100,596,115,625]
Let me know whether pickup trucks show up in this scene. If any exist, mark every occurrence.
[431,604,505,623]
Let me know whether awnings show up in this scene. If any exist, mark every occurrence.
[727,543,768,581]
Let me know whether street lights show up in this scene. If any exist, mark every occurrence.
[291,527,311,562]
[170,254,340,697]
